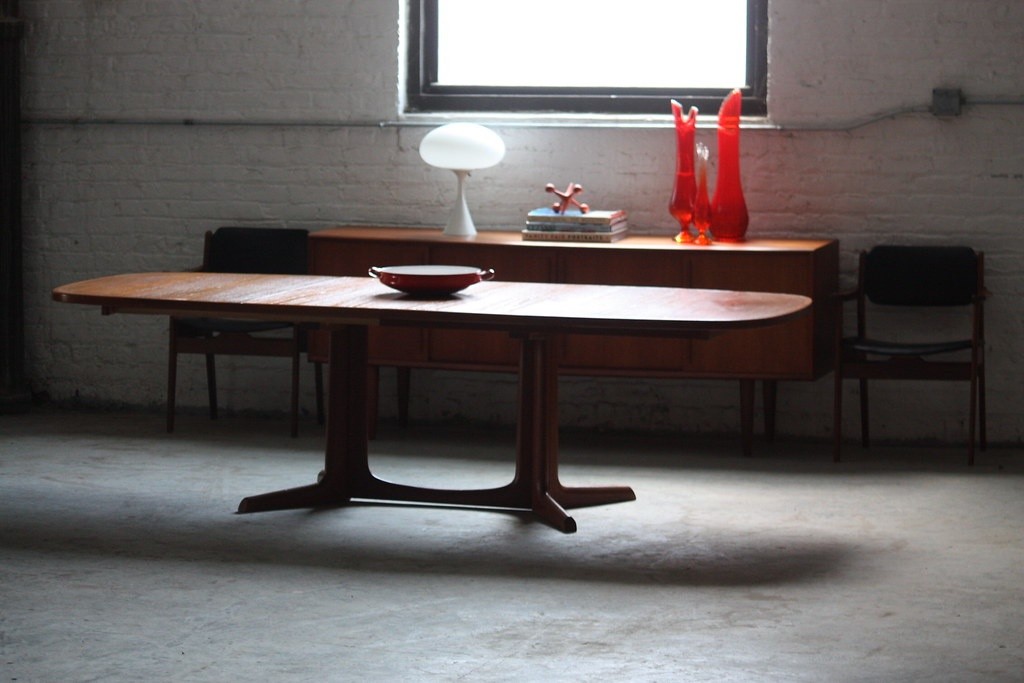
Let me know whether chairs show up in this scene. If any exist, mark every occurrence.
[825,241,990,468]
[166,225,324,440]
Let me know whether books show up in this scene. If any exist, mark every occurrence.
[523,230,627,243]
[528,207,627,225]
[526,221,628,231]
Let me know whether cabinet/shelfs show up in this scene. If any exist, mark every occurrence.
[311,229,843,445]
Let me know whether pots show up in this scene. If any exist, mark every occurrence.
[368,266,495,295]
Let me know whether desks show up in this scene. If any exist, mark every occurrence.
[55,273,812,534]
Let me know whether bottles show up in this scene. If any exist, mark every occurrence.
[667,99,698,243]
[694,143,712,246]
[707,90,749,239]
